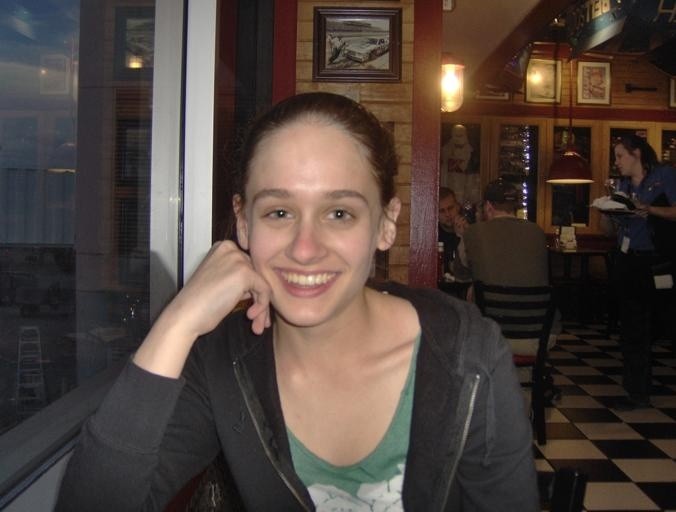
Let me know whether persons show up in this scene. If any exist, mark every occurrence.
[325,34,351,65]
[54,90,543,511]
[438,184,468,276]
[453,179,559,358]
[442,124,477,174]
[607,133,675,403]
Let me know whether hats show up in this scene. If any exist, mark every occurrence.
[485,180,520,202]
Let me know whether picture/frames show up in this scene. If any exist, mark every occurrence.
[113,5,153,82]
[475,87,510,100]
[115,116,151,186]
[312,5,403,84]
[525,58,562,102]
[441,114,676,233]
[577,60,610,105]
[118,196,150,290]
[669,77,676,109]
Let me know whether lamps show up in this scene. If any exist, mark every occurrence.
[546,61,593,184]
[441,51,466,113]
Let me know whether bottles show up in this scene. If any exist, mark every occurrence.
[437,241,446,284]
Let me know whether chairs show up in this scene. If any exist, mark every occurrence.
[537,465,586,512]
[475,283,558,445]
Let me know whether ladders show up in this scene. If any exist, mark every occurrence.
[15,326,46,414]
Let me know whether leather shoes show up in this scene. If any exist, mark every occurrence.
[627,391,650,408]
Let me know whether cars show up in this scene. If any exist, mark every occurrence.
[343,35,389,63]
